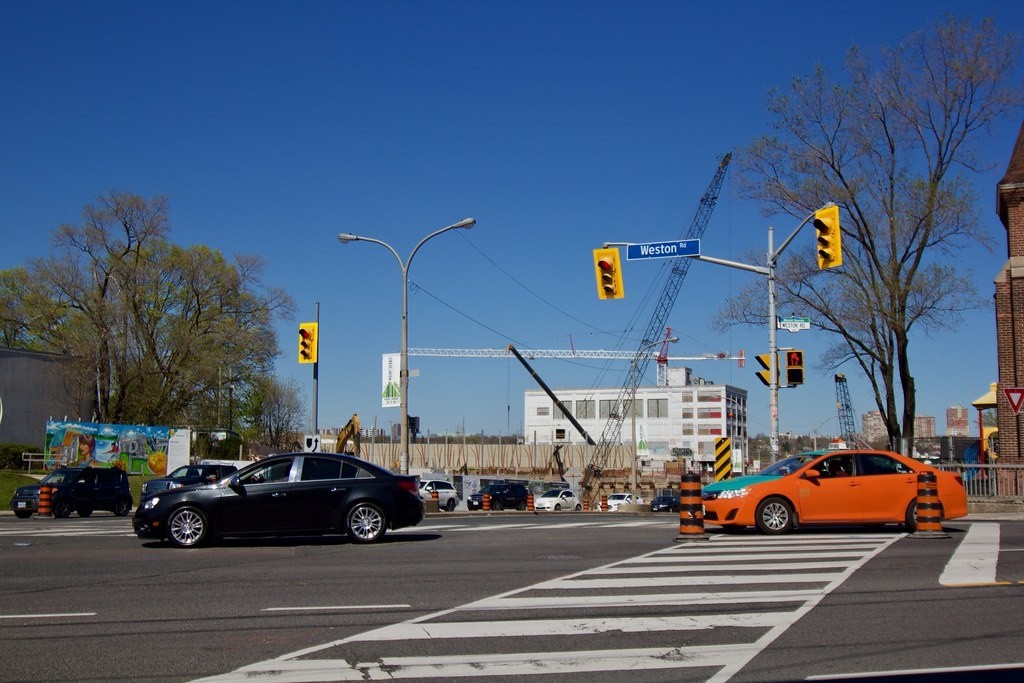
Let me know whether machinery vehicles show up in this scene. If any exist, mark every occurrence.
[336,413,362,459]
[834,373,865,451]
[570,150,739,515]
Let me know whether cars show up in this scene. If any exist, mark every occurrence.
[649,494,682,512]
[131,452,428,549]
[597,492,645,512]
[703,449,972,538]
[536,490,581,511]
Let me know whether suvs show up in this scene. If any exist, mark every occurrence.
[467,482,528,512]
[11,466,134,517]
[417,479,460,512]
[137,465,239,505]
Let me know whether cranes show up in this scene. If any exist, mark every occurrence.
[413,327,749,389]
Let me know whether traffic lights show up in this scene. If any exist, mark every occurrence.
[593,248,624,301]
[786,351,804,384]
[298,322,321,364]
[815,206,843,270]
[754,353,783,389]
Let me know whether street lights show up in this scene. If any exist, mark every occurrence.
[812,414,849,450]
[338,216,478,476]
[630,337,679,515]
